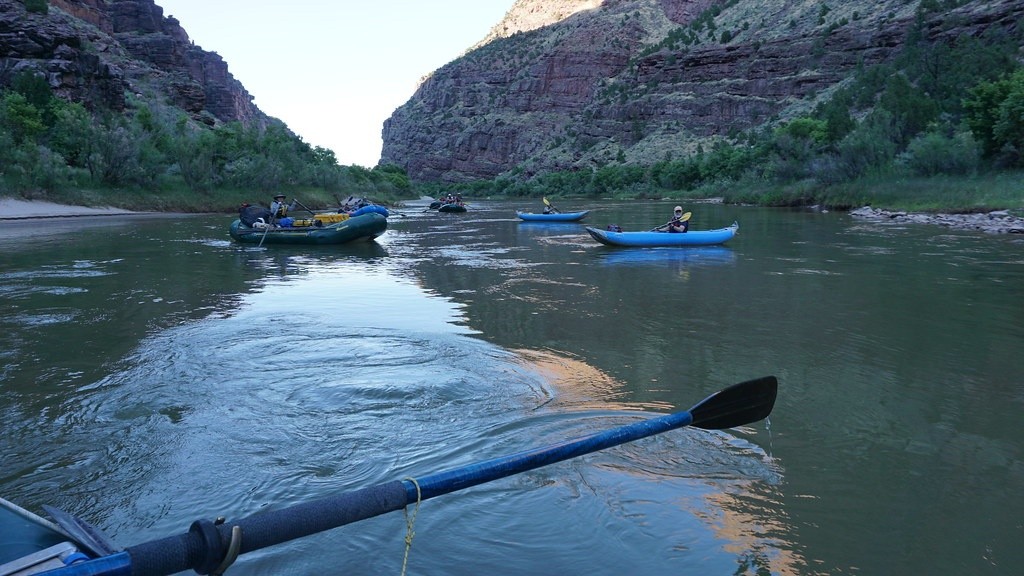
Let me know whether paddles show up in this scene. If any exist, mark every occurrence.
[292,198,316,215]
[542,196,561,214]
[258,203,280,248]
[27,373,779,576]
[650,211,692,231]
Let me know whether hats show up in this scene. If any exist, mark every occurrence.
[674,205,683,213]
[273,193,287,200]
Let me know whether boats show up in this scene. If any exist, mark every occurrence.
[439,202,468,213]
[431,197,447,209]
[1,495,138,575]
[514,210,591,222]
[229,198,387,247]
[340,198,389,219]
[585,220,740,247]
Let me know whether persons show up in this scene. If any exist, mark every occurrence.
[440,193,462,204]
[359,196,368,208]
[543,204,555,214]
[269,195,296,223]
[656,206,688,233]
